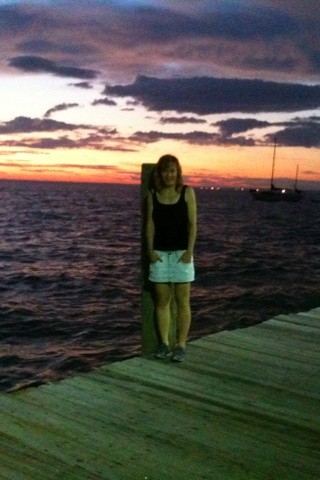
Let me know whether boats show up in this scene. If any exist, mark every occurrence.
[249,187,307,203]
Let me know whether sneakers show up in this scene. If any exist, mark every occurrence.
[155,343,173,359]
[172,345,185,361]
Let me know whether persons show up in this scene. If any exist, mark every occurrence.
[146,154,198,362]
